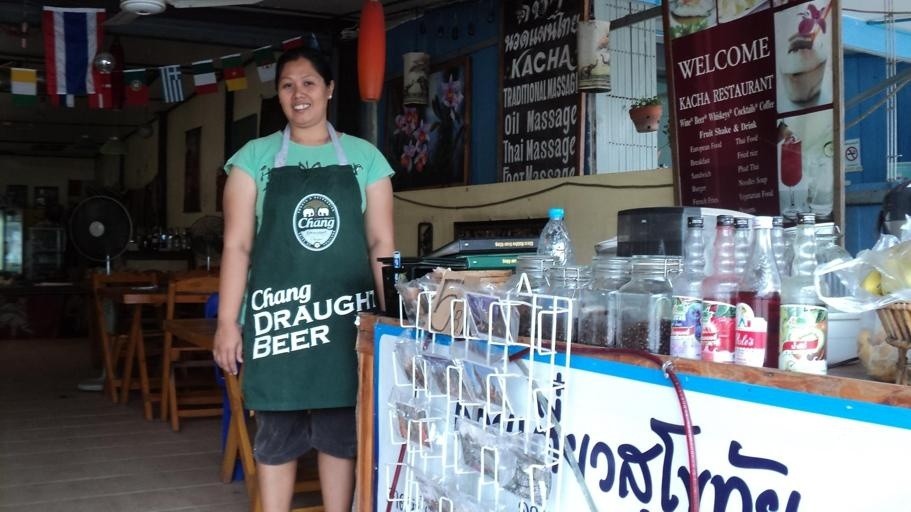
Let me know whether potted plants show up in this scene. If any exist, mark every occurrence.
[629,96,664,132]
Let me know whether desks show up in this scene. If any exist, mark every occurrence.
[163,318,328,512]
[96,287,222,421]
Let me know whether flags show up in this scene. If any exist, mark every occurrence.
[45,7,105,96]
[88,70,113,110]
[254,45,282,83]
[191,60,219,97]
[12,67,38,109]
[159,63,185,102]
[223,54,251,93]
[283,37,304,53]
[122,68,149,108]
[49,96,74,110]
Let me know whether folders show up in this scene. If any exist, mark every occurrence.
[455,255,541,270]
[428,239,539,257]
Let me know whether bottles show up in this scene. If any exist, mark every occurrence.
[537,265,593,343]
[577,255,631,348]
[669,216,709,361]
[615,255,685,355]
[700,215,741,365]
[778,213,828,376]
[733,218,751,285]
[536,208,575,286]
[781,221,855,297]
[733,216,782,369]
[770,217,785,283]
[514,255,560,339]
[151,225,190,253]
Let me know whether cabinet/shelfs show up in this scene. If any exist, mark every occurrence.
[350,311,911,512]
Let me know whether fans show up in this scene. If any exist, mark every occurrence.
[67,196,133,391]
[36,0,261,23]
[188,215,224,271]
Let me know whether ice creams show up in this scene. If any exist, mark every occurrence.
[779,33,827,104]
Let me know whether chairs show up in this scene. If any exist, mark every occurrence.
[203,292,257,481]
[161,275,219,431]
[93,270,162,404]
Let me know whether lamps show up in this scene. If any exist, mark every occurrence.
[358,1,386,102]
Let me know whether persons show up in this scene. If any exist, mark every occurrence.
[211,48,397,512]
